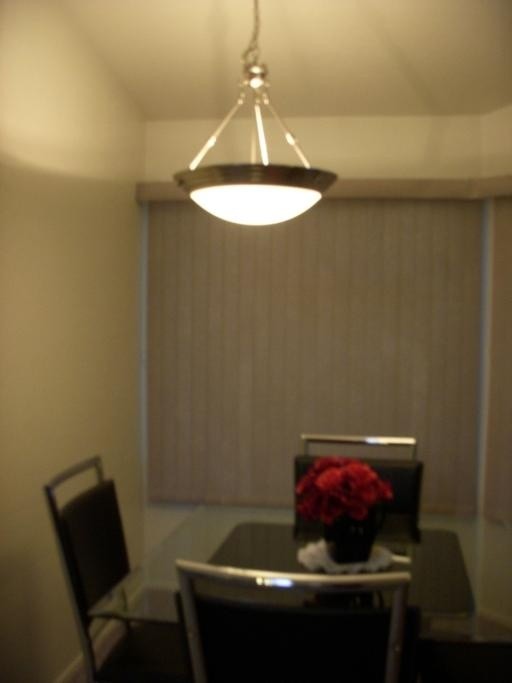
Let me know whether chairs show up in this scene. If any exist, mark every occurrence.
[293,433,423,528]
[174,559,413,683]
[42,454,194,683]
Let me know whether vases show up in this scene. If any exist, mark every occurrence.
[321,524,380,564]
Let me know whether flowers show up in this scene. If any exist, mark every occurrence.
[294,454,394,524]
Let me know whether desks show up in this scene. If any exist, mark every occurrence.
[86,504,512,644]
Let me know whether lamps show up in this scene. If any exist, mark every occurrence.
[173,0,339,226]
